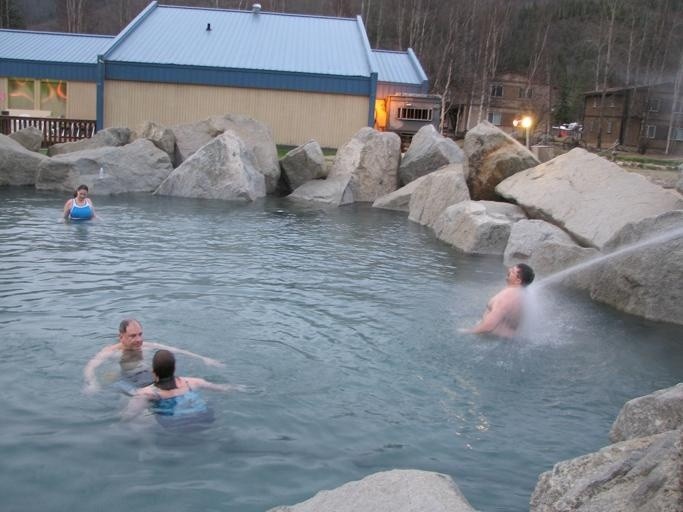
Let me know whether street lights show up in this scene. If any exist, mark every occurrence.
[521,114,533,149]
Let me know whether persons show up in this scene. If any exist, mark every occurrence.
[471,262,537,342]
[60,185,96,222]
[82,316,224,394]
[113,349,239,434]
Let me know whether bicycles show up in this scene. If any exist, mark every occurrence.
[561,129,587,150]
